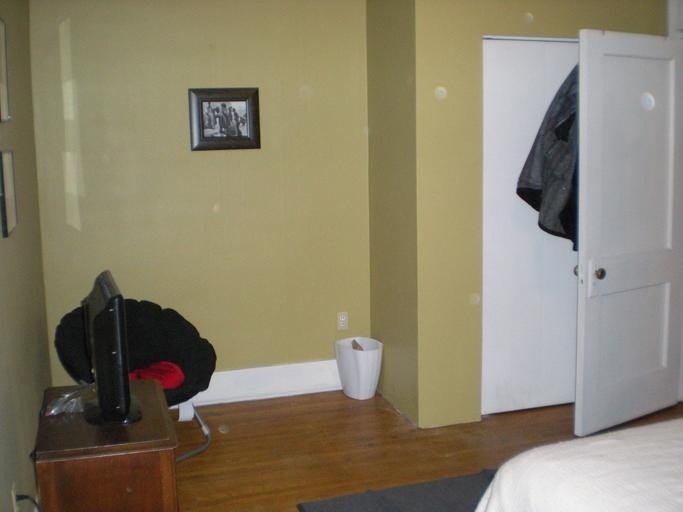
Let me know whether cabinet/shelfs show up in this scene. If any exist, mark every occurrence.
[34,378,178,512]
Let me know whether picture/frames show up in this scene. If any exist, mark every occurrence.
[0,19,10,121]
[188,87,261,151]
[0,150,18,238]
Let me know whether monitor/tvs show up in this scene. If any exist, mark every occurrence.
[81,269,142,427]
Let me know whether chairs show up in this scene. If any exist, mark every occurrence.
[55,299,217,463]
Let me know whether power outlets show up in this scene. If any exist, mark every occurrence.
[336,312,349,330]
[11,484,17,512]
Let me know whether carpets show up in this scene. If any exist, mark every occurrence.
[297,469,496,512]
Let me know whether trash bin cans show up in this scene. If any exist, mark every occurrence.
[333,336,383,401]
